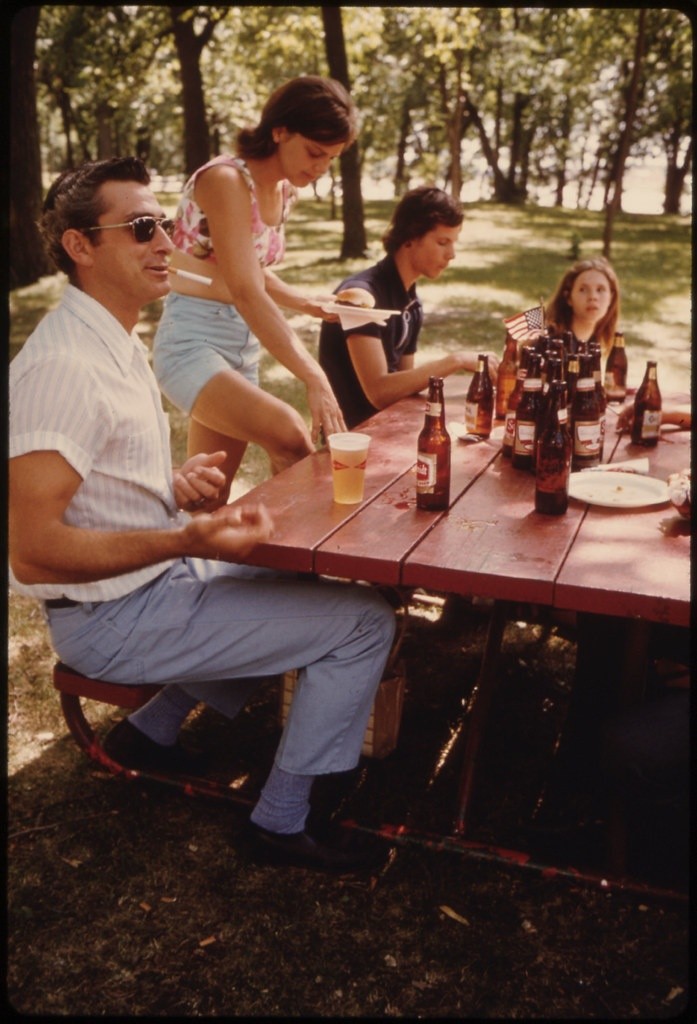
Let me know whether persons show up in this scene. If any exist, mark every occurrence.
[153,74,361,513]
[548,257,627,385]
[318,187,500,430]
[602,400,691,882]
[9,155,409,875]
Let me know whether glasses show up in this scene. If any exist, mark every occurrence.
[83,218,175,244]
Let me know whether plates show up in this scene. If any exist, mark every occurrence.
[311,301,402,319]
[567,472,671,508]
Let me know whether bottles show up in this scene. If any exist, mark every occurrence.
[503,332,606,470]
[605,332,628,403]
[631,361,662,447]
[496,340,518,420]
[465,354,494,435]
[536,381,572,514]
[417,377,451,510]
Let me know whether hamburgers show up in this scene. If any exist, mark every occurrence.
[335,287,375,309]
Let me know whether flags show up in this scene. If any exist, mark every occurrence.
[502,305,544,340]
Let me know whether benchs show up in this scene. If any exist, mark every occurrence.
[52,666,159,709]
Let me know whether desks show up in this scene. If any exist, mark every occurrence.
[195,373,692,904]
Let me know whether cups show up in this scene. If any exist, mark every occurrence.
[327,433,371,504]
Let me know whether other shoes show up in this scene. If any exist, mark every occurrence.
[237,816,372,872]
[95,717,215,776]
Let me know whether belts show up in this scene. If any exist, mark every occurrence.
[45,598,77,609]
